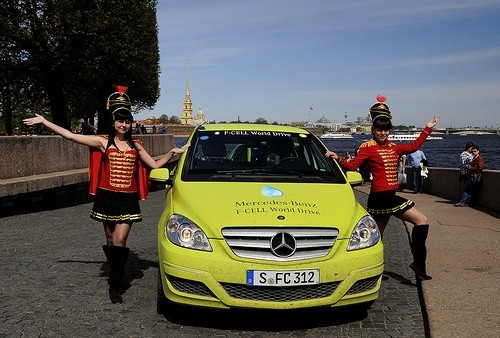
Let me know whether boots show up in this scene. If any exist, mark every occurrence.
[409,224,433,280]
[109,245,126,304]
[102,244,130,295]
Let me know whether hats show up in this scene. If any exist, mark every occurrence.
[106,85,132,115]
[368,94,392,121]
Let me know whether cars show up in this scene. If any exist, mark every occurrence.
[149,123,383,309]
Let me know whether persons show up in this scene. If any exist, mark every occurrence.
[397,153,407,191]
[21,91,186,289]
[344,151,350,160]
[459,142,476,189]
[325,102,440,282]
[406,148,427,194]
[135,123,147,134]
[161,127,166,134]
[454,148,484,207]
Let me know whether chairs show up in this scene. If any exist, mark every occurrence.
[202,140,228,169]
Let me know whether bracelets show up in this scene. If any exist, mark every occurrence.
[422,130,430,135]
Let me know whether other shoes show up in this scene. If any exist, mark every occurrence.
[454,203,463,207]
[460,204,468,207]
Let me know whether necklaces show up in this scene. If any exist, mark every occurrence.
[119,144,129,157]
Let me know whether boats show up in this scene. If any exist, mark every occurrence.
[319,131,353,141]
[460,132,468,137]
[363,135,442,141]
